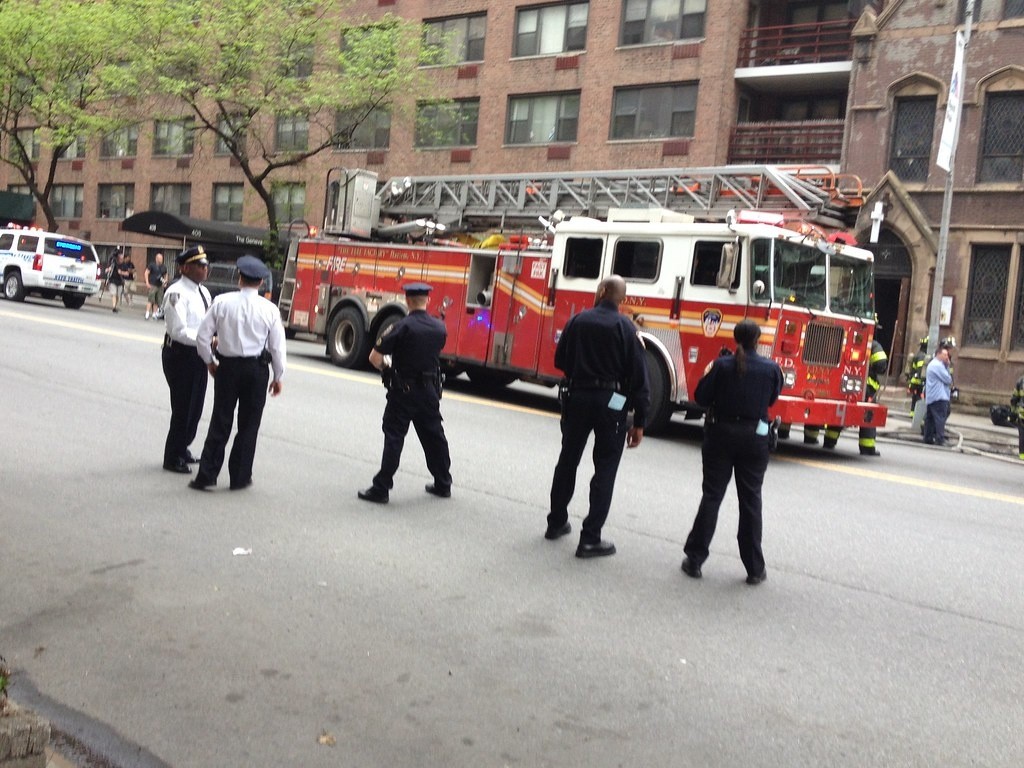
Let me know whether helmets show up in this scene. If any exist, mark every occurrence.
[948,337,955,344]
[920,336,929,344]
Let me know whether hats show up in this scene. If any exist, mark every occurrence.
[178,244,208,265]
[405,283,431,296]
[237,255,270,281]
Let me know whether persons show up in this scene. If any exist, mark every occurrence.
[680,318,784,586]
[920,336,957,446]
[822,312,887,456]
[357,283,454,504]
[778,416,819,445]
[105,253,168,320]
[1010,375,1024,460]
[188,256,288,493]
[162,244,219,474]
[905,335,929,418]
[544,275,650,559]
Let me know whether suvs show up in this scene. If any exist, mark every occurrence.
[0,228,103,309]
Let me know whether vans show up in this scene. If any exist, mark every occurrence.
[160,262,282,316]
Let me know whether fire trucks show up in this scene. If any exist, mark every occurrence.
[274,164,889,433]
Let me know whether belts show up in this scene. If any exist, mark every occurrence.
[573,381,620,390]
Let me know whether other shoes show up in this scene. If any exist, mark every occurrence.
[163,465,192,473]
[425,483,451,497]
[188,457,201,462]
[249,479,252,484]
[191,479,217,486]
[860,451,880,455]
[682,558,701,577]
[746,568,765,584]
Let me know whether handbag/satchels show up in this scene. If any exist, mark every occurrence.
[991,405,1018,426]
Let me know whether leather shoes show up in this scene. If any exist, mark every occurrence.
[545,522,571,539]
[358,487,389,502]
[575,541,614,557]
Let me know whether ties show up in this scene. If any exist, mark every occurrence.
[199,287,208,314]
